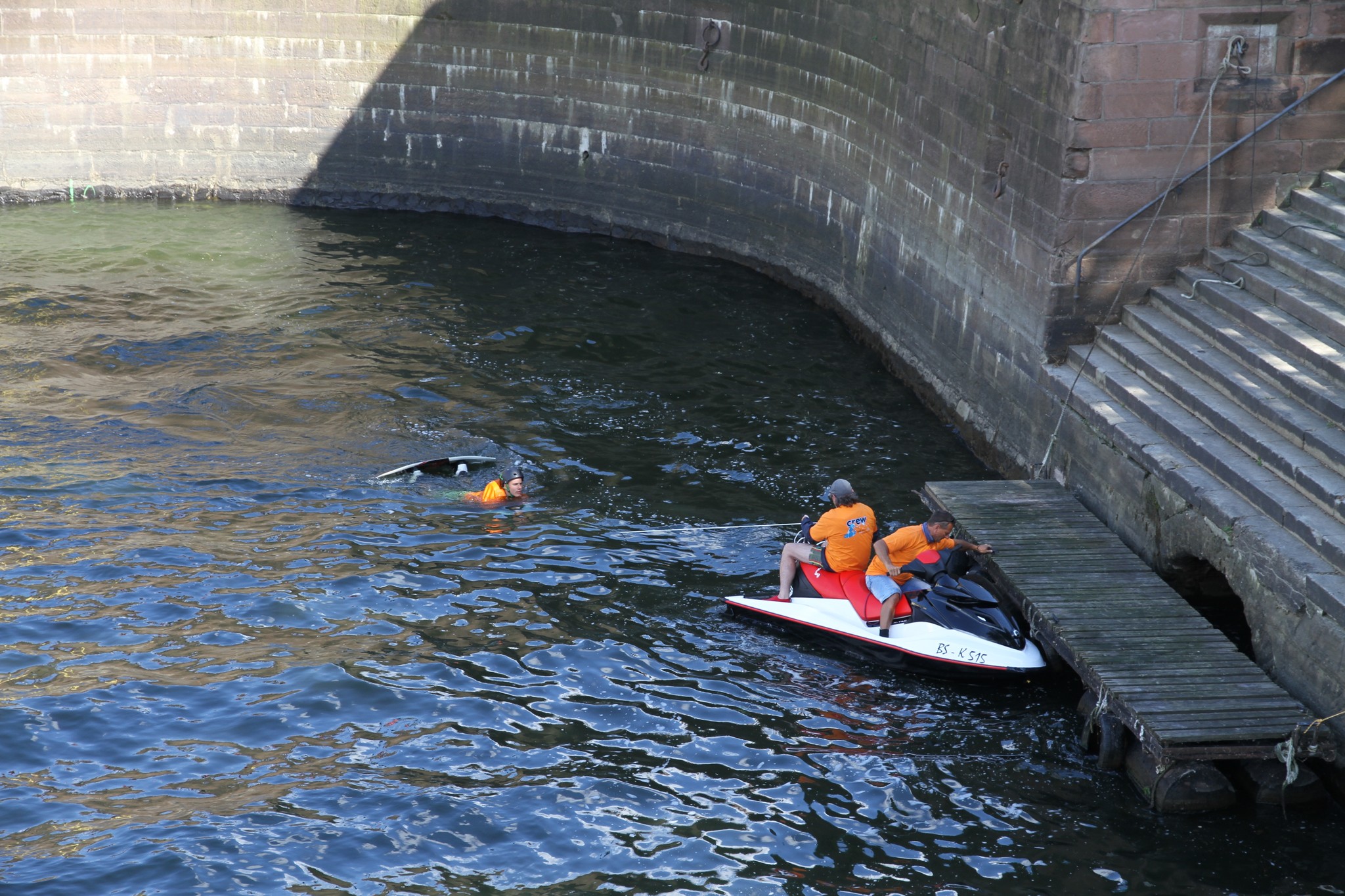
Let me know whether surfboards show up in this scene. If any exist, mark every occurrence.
[376,456,496,479]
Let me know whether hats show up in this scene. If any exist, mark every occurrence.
[829,479,852,496]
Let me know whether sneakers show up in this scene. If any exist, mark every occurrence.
[408,467,421,473]
[454,461,468,476]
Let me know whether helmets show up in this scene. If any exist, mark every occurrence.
[500,467,523,487]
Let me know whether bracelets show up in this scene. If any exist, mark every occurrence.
[977,545,980,550]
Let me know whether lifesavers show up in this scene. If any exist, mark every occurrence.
[1078,709,1128,772]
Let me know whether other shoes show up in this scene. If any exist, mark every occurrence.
[763,595,790,602]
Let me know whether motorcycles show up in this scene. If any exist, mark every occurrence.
[724,544,1046,687]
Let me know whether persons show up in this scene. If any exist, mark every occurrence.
[433,467,530,505]
[759,478,879,603]
[864,509,993,638]
